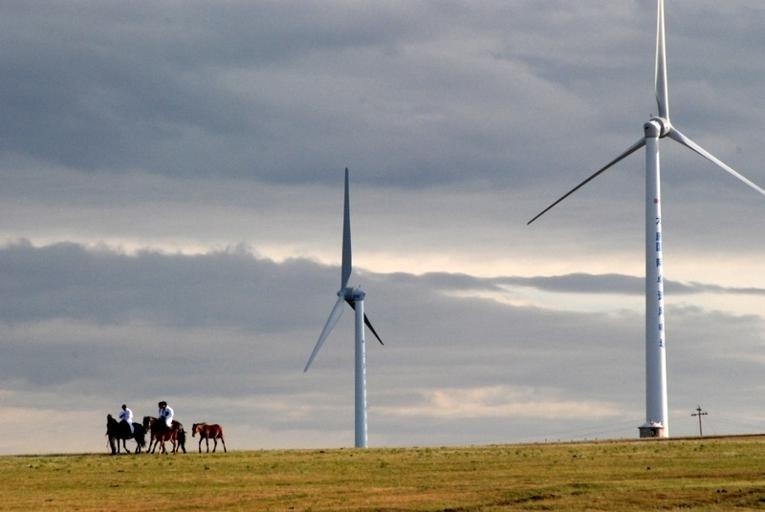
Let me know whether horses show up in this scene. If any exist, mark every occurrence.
[143,413,189,455]
[191,422,226,453]
[108,416,145,454]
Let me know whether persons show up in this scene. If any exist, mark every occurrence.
[117,404,135,434]
[159,400,175,432]
[158,401,164,417]
